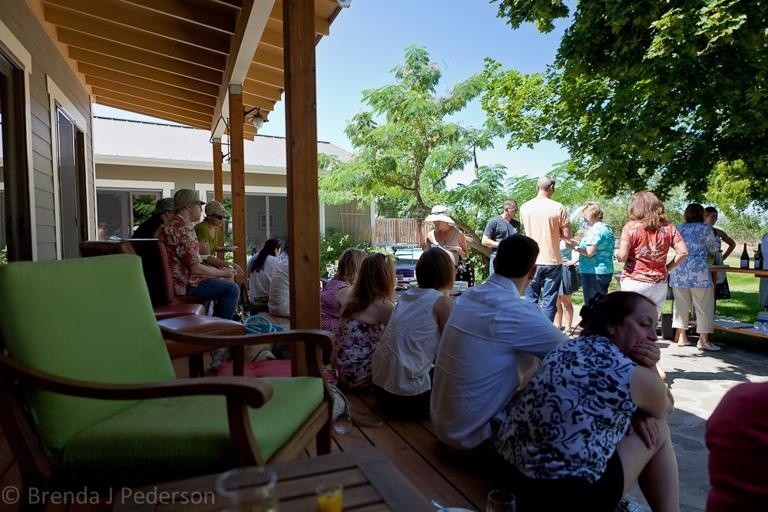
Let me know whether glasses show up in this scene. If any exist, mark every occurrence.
[429,210,446,215]
[213,214,223,220]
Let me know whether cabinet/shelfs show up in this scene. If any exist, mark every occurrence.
[672,263,768,345]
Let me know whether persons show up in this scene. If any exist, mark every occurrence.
[429,234,574,478]
[130,189,246,375]
[704,378,768,512]
[267,241,290,318]
[96,220,121,241]
[247,237,283,309]
[759,232,768,311]
[251,243,264,257]
[329,252,399,395]
[480,174,737,351]
[491,292,679,512]
[370,247,458,422]
[416,203,475,287]
[320,248,364,335]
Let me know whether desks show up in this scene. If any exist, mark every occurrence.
[107,445,445,512]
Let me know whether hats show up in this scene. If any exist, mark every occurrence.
[205,200,226,218]
[174,188,206,210]
[424,204,456,227]
[152,197,175,214]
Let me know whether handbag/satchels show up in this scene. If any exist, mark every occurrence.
[454,254,475,296]
[324,383,384,435]
[243,314,285,363]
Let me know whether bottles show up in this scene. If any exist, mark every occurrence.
[754,243,763,269]
[740,244,749,269]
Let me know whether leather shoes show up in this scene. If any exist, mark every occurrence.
[697,339,721,352]
[677,339,696,347]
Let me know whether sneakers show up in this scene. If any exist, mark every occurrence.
[562,330,573,336]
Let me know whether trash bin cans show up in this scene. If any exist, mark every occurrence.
[662,314,676,339]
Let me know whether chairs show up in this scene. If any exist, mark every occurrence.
[1,248,337,512]
[77,232,250,378]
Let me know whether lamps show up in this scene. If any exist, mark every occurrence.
[221,149,231,165]
[244,103,269,130]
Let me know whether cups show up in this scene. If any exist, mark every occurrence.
[314,483,343,511]
[214,466,281,512]
[487,489,517,511]
